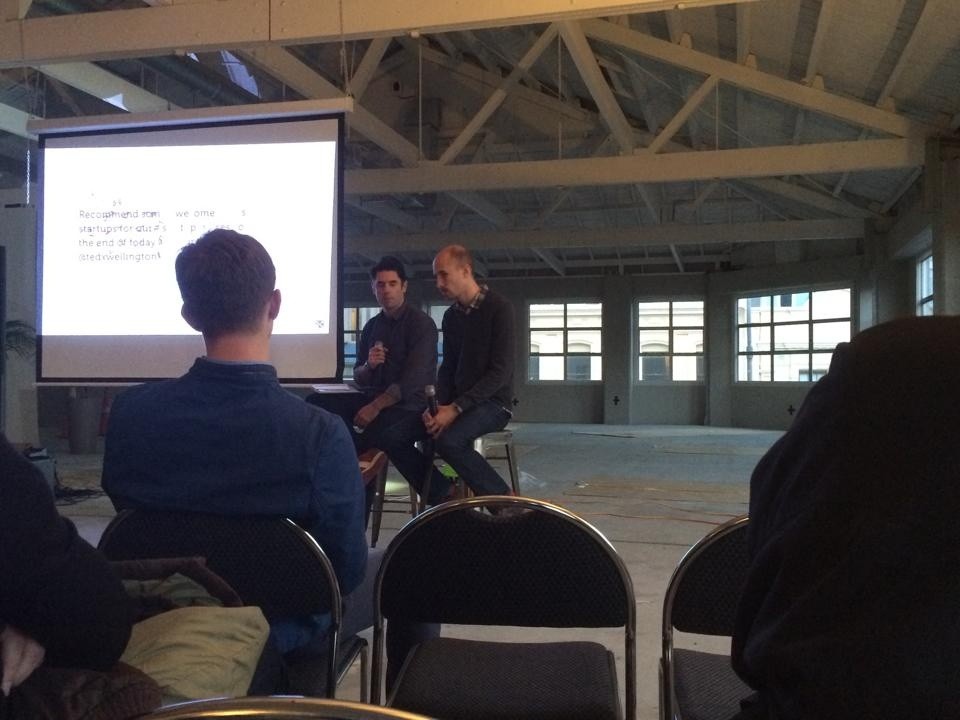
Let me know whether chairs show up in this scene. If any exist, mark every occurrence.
[97,507,368,703]
[371,495,638,720]
[658,514,758,720]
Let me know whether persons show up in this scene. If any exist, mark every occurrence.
[101,227,387,645]
[0,433,136,720]
[731,314,959,720]
[349,246,527,520]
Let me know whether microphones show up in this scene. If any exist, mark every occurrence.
[426,384,438,416]
[374,341,385,384]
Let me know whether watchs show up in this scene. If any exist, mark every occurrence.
[364,360,372,372]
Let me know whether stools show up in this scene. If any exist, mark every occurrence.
[371,430,520,547]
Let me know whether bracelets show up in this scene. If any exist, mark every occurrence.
[452,402,461,415]
